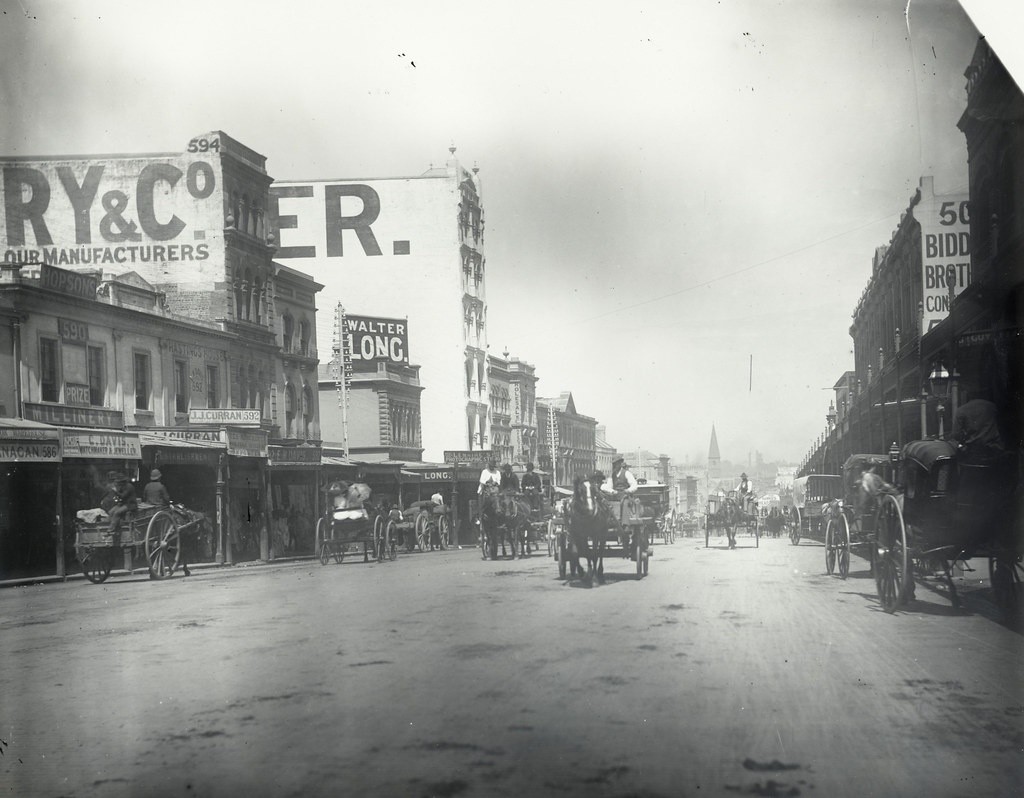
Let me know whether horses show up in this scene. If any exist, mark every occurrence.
[721,498,740,550]
[499,493,539,560]
[148,502,213,578]
[854,466,961,609]
[482,487,506,559]
[364,505,404,561]
[562,475,610,587]
[820,498,847,545]
[765,506,781,537]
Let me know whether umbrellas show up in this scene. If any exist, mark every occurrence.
[347,483,372,502]
[322,478,349,494]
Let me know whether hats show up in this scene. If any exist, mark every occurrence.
[525,463,535,468]
[114,473,128,481]
[612,456,624,463]
[150,469,162,480]
[488,460,498,468]
[740,473,748,477]
[501,463,512,469]
[391,504,399,509]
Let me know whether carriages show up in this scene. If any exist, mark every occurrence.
[314,479,452,566]
[553,471,659,588]
[476,440,1023,636]
[73,497,214,585]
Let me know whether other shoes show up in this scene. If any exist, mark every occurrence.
[624,526,632,534]
[103,531,120,537]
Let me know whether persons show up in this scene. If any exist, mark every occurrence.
[477,461,500,494]
[783,506,788,514]
[955,387,1006,452]
[431,488,444,505]
[99,471,138,535]
[143,468,171,509]
[500,463,519,493]
[334,491,365,508]
[389,504,403,520]
[521,462,542,493]
[600,456,637,533]
[861,464,896,508]
[759,507,768,521]
[734,472,753,513]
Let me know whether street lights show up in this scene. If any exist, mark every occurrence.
[928,358,950,441]
